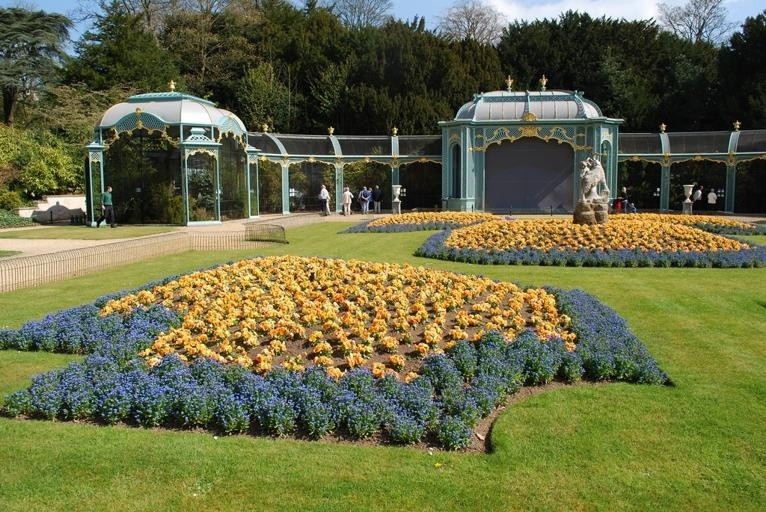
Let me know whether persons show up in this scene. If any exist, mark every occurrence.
[629,202,636,212]
[95,185,115,228]
[692,185,705,215]
[619,186,629,214]
[707,188,718,210]
[318,183,382,215]
